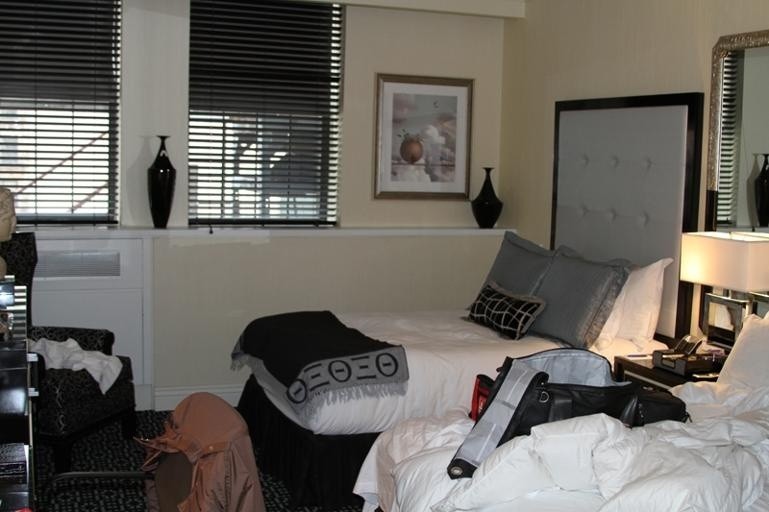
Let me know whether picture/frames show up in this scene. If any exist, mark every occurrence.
[372,71,476,201]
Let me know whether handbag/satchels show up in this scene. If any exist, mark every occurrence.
[447,347,637,486]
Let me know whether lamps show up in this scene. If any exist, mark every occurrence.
[672,224,769,355]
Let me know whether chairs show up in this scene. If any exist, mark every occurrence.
[44,389,251,511]
[0,231,141,459]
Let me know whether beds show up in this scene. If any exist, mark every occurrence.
[347,380,769,510]
[224,294,684,510]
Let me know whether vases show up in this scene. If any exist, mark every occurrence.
[753,150,769,228]
[468,163,504,231]
[144,133,184,229]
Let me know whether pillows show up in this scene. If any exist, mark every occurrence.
[614,253,676,351]
[714,313,769,402]
[759,309,769,323]
[460,278,545,340]
[590,269,632,350]
[465,231,641,352]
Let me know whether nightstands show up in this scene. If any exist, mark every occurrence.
[610,351,725,397]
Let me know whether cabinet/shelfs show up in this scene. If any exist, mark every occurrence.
[0,283,42,511]
